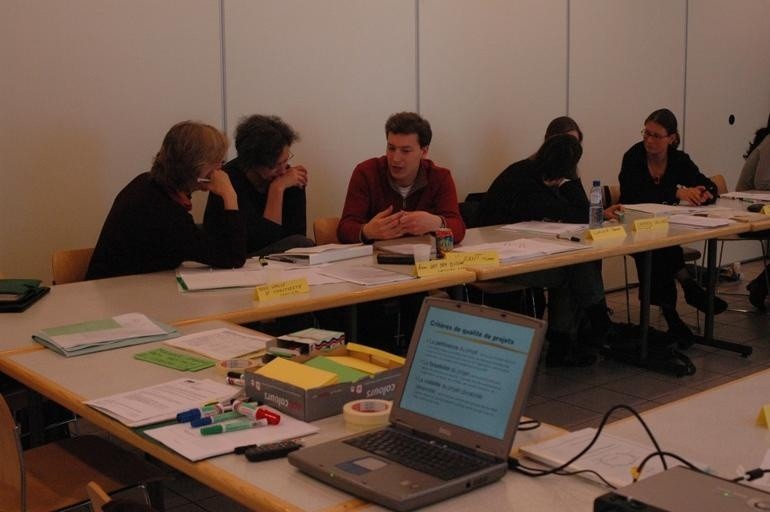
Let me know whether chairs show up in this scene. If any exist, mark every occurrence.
[86,480,117,512]
[0,392,179,511]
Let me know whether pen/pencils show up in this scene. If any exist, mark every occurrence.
[198,176,211,183]
[701,183,715,194]
[556,234,580,242]
[265,256,296,264]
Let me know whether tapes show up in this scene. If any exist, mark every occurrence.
[216,358,257,377]
[343,398,392,426]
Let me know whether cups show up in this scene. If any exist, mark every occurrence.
[412,244,431,262]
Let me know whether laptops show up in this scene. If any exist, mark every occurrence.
[288,298,549,511]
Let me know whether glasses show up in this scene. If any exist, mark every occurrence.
[641,129,670,139]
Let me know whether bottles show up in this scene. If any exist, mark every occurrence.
[588,179,604,227]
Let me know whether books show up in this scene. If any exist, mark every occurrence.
[620,200,732,217]
[721,189,770,203]
[454,237,591,264]
[174,240,416,295]
[30,311,180,357]
[499,217,588,236]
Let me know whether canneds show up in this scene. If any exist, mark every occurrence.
[436,227,454,258]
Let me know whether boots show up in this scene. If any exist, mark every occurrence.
[589,297,635,336]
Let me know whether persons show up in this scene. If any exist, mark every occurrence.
[734,114,770,311]
[527,116,610,327]
[618,109,727,348]
[475,132,612,366]
[335,112,467,350]
[84,121,246,281]
[203,114,309,259]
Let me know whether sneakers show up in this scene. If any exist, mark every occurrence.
[667,312,697,349]
[746,281,767,312]
[544,349,597,369]
[685,286,729,316]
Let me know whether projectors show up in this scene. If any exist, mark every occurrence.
[593,464,770,512]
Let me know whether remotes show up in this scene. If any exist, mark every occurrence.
[246,441,298,462]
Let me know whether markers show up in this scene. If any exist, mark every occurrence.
[226,378,244,386]
[176,397,281,436]
[227,371,245,379]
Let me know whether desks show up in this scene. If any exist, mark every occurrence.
[349,364,769,512]
[1,312,569,511]
[373,190,768,368]
[1,253,476,450]
